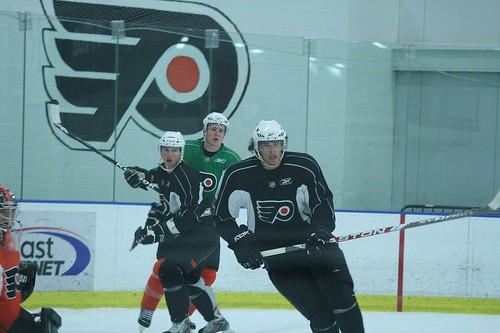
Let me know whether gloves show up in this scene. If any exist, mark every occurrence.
[135,207,168,245]
[227,228,264,270]
[305,229,331,257]
[122,166,150,189]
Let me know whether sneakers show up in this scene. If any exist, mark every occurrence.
[137,307,153,333]
[197,316,235,333]
[189,321,196,333]
[163,317,192,333]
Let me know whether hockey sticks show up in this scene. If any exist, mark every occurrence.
[50,98,165,196]
[259,189,500,257]
[128,202,156,254]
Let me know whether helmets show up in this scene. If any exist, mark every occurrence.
[203,112,230,135]
[248,120,287,163]
[0,184,18,242]
[159,131,185,157]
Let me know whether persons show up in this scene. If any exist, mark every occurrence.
[121,112,243,333]
[210,119,366,333]
[0,188,61,332]
